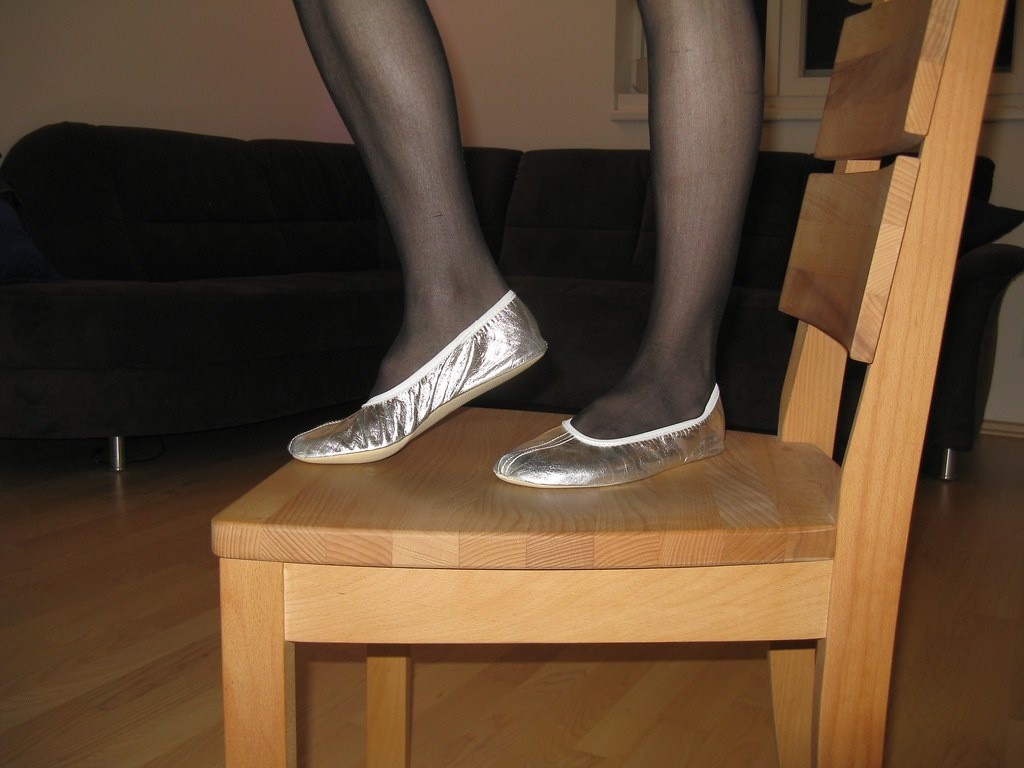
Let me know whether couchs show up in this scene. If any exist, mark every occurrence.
[0,121,1024,482]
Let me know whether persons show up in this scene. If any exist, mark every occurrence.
[289,0,764,489]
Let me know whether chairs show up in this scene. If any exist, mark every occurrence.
[212,0,1007,767]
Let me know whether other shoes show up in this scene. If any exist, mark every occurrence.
[287,288,548,464]
[492,382,726,488]
